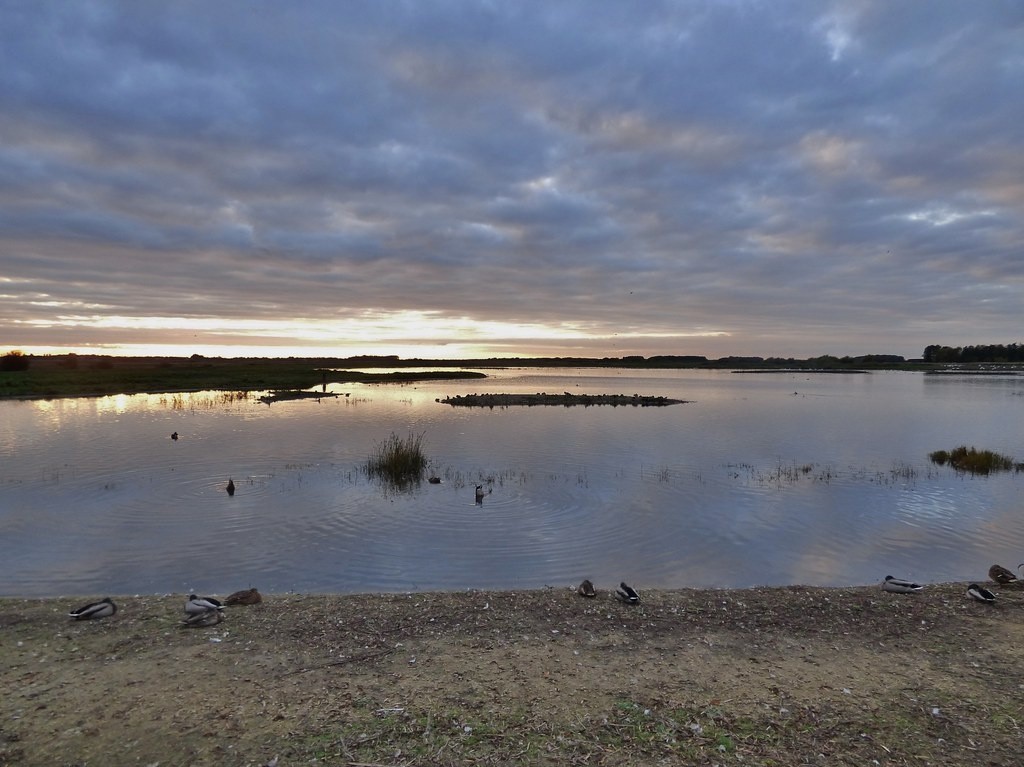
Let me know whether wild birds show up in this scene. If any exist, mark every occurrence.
[989,565,1017,584]
[223,587,262,606]
[68,597,118,620]
[184,594,228,614]
[578,579,596,597]
[884,575,923,593]
[615,582,640,604]
[966,583,999,603]
[226,480,235,496]
[171,432,179,440]
[476,485,484,500]
